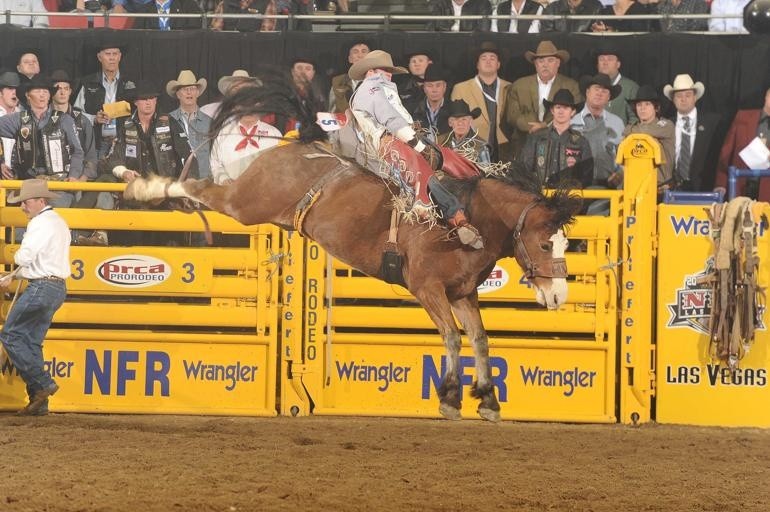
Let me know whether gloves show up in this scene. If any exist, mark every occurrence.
[408,133,432,162]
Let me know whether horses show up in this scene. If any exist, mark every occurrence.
[122,64,585,423]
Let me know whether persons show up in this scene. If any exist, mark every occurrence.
[1,42,374,247]
[349,51,486,250]
[397,42,722,217]
[0,1,770,36]
[713,85,770,206]
[1,179,71,416]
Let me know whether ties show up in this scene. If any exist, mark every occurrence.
[678,117,691,182]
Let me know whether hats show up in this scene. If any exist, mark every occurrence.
[579,74,621,100]
[6,178,62,206]
[275,36,455,82]
[625,84,666,113]
[593,43,621,56]
[0,33,128,101]
[121,70,262,101]
[525,41,569,64]
[543,89,583,113]
[439,99,482,119]
[465,42,503,68]
[663,74,705,101]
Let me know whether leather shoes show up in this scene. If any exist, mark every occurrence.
[29,382,58,411]
[456,220,485,250]
[16,398,49,415]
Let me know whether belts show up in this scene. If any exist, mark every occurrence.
[43,275,65,283]
